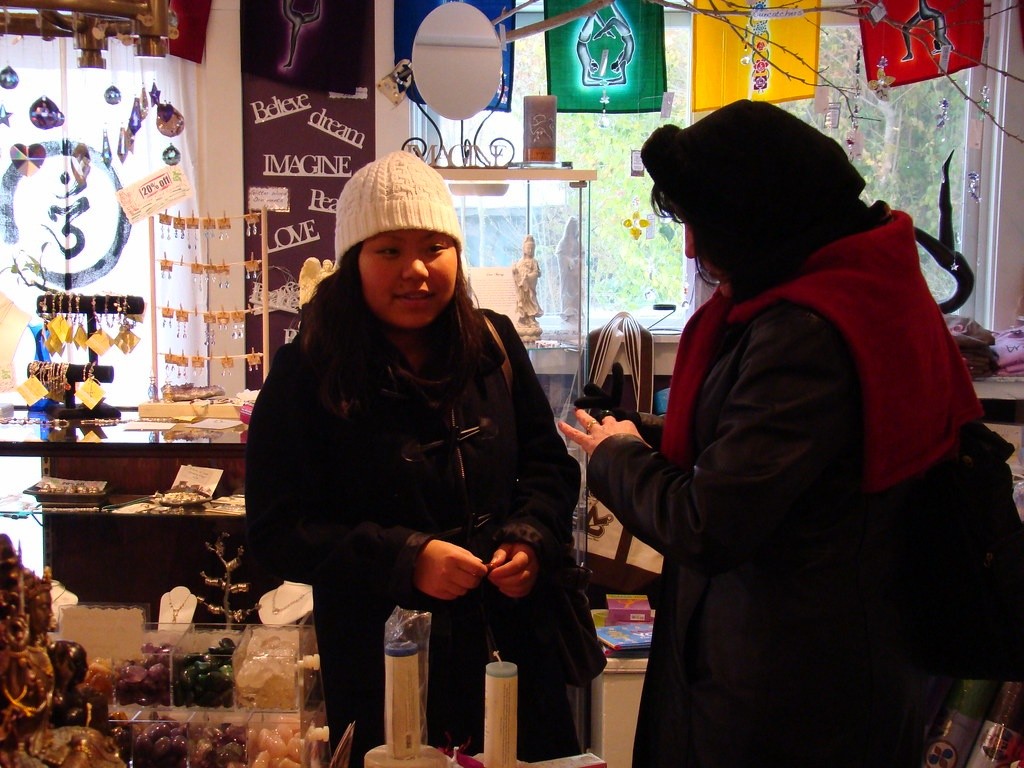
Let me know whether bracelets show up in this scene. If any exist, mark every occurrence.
[29,360,101,404]
[39,290,137,331]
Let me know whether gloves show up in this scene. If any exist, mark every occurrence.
[573,363,664,445]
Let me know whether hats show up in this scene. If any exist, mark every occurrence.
[642,97,864,300]
[334,151,463,269]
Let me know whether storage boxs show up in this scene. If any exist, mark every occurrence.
[108,624,327,768]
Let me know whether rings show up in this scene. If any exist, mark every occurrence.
[585,419,598,433]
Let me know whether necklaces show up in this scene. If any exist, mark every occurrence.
[168,592,191,625]
[272,589,312,615]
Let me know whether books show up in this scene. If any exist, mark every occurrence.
[592,622,654,659]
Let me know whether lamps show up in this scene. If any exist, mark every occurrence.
[0,0,170,68]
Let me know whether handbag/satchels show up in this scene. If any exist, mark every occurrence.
[527,535,607,687]
[916,421,1023,683]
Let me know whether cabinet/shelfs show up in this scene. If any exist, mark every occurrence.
[0,417,247,528]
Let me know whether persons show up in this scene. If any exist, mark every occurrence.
[555,216,587,326]
[511,234,545,343]
[554,97,976,768]
[244,152,583,768]
[0,532,125,768]
